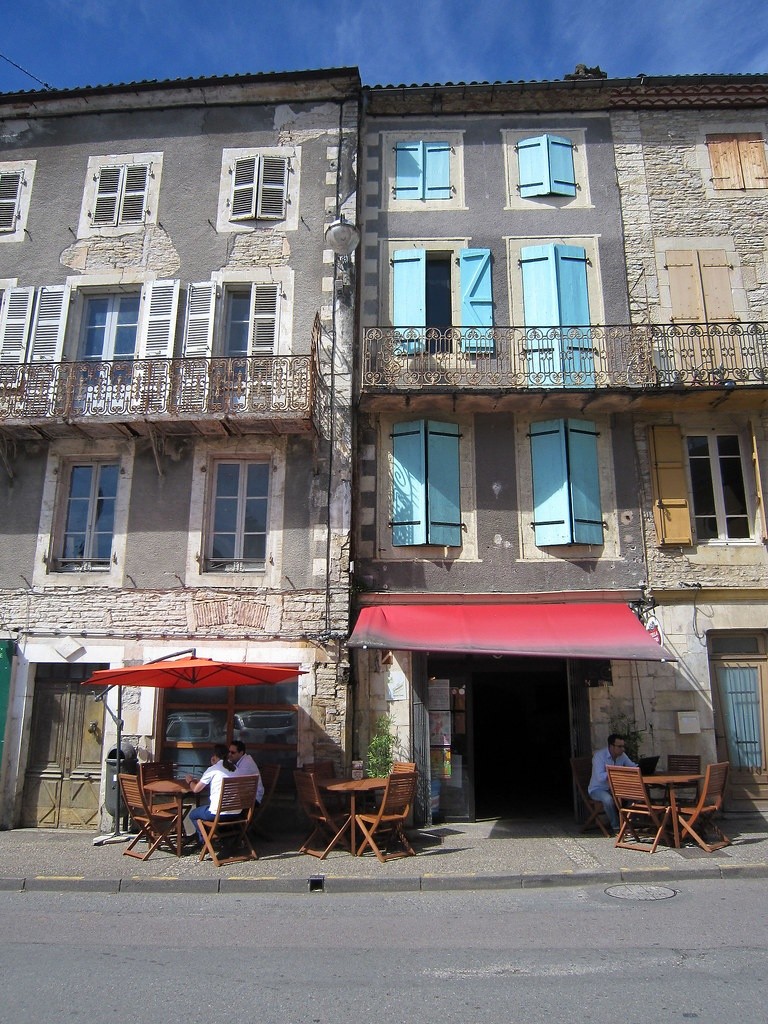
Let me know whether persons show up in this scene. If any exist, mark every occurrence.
[588,733,638,831]
[186,740,264,848]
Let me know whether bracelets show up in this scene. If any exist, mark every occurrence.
[189,781,193,786]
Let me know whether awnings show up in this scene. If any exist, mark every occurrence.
[347,603,677,663]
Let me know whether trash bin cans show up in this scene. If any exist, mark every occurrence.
[105,741,138,817]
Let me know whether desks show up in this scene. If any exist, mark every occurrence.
[144,779,210,858]
[643,774,705,848]
[317,778,387,855]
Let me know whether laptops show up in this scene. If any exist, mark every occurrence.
[638,756,661,776]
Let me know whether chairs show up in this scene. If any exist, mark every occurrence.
[194,773,259,867]
[118,772,179,862]
[663,754,702,815]
[570,757,613,837]
[302,760,350,813]
[293,770,349,860]
[356,771,419,863]
[676,761,732,853]
[139,762,192,849]
[375,762,416,815]
[605,764,672,854]
[247,762,282,837]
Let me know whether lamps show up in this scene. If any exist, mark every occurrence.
[323,213,360,288]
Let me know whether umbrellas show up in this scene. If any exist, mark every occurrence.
[81,656,309,833]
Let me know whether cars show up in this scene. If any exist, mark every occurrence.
[220,713,297,773]
[166,708,217,750]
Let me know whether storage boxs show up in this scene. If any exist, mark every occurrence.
[352,761,364,770]
[445,754,469,788]
[351,771,364,779]
[428,712,453,746]
[430,747,452,779]
[429,687,451,710]
